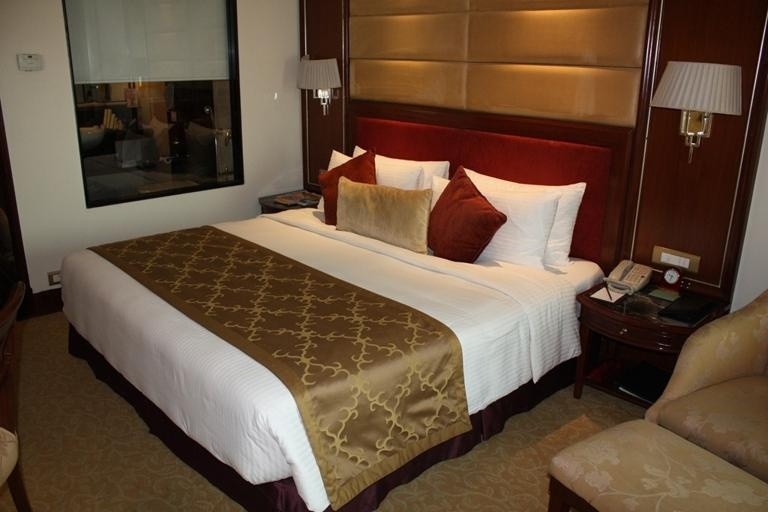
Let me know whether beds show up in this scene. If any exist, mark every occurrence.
[61,120,612,512]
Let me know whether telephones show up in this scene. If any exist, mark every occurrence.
[602,260,653,296]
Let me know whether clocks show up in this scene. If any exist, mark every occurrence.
[664,269,680,284]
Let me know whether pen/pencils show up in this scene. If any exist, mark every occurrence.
[603,280,612,301]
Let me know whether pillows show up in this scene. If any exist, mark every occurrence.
[316,145,587,272]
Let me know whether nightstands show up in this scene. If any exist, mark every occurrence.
[574,283,719,410]
[258,190,321,214]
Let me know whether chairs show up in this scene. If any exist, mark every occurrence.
[644,289,768,483]
[0,281,32,512]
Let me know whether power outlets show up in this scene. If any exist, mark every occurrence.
[48,270,60,286]
[652,246,701,274]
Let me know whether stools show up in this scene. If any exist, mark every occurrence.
[548,420,768,512]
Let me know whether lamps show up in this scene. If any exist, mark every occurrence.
[296,55,341,115]
[649,61,742,162]
